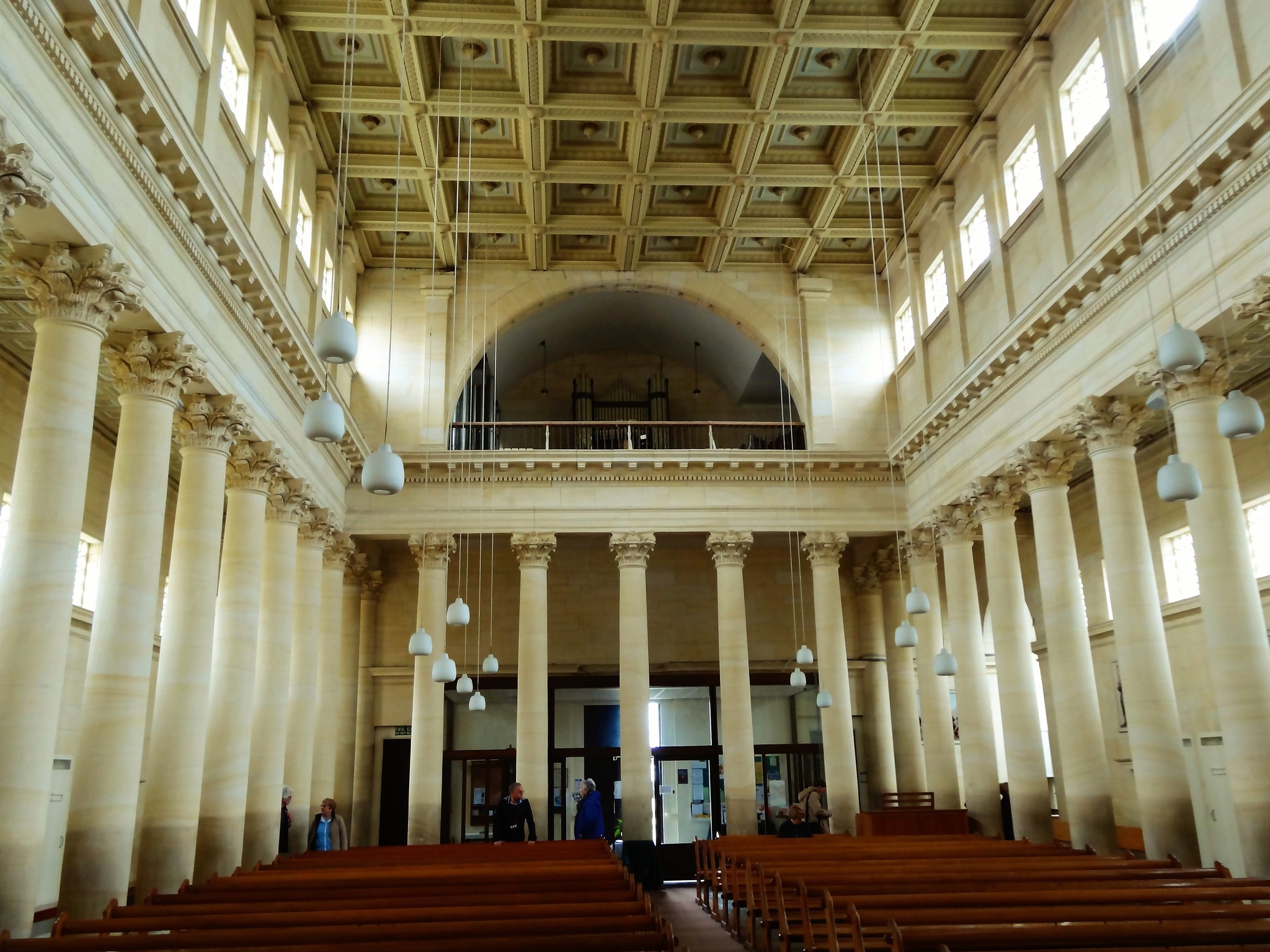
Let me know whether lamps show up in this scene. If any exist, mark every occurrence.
[778,1,1263,707]
[305,0,498,710]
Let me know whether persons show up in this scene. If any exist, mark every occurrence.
[573,778,604,840]
[278,785,292,858]
[307,798,347,851]
[777,806,814,838]
[798,779,832,834]
[492,783,537,846]
[998,782,1015,841]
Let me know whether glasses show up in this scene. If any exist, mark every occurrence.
[518,790,524,794]
[288,796,292,798]
[321,804,325,807]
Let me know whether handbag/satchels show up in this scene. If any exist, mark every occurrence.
[808,822,823,834]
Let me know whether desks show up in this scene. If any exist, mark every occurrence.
[852,808,970,836]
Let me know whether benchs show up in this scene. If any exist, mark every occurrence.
[0,831,1270,952]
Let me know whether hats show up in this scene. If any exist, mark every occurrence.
[789,805,805,819]
